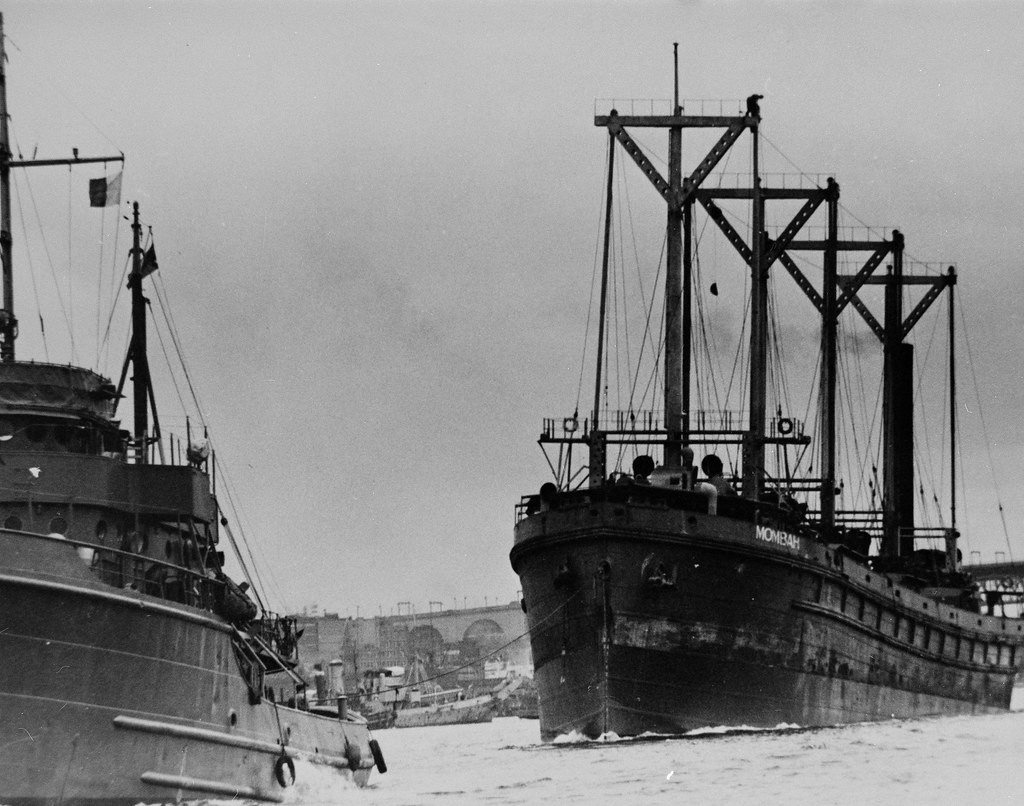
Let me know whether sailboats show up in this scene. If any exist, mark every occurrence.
[2,28,384,803]
[500,38,1021,745]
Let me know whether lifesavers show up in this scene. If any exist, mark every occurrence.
[778,419,794,434]
[563,418,579,432]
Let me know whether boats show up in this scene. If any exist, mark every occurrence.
[289,642,538,740]
